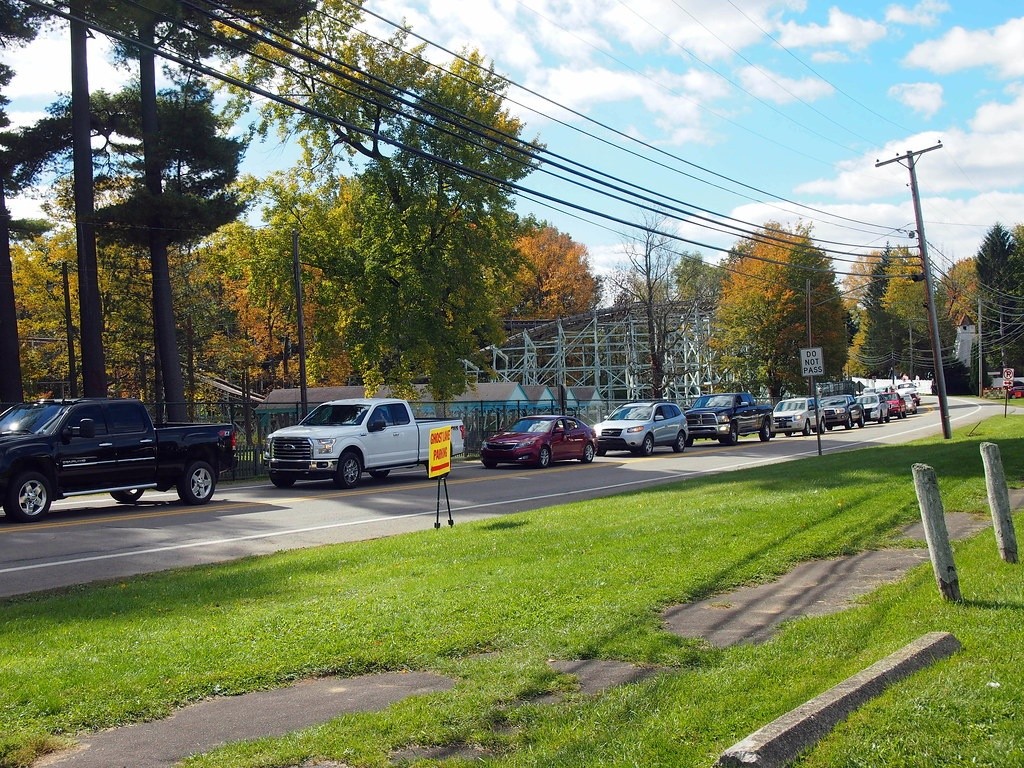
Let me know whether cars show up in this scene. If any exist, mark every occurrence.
[1008,381,1024,399]
[883,393,907,419]
[480,416,598,469]
[900,394,917,415]
[855,394,890,424]
[896,383,921,405]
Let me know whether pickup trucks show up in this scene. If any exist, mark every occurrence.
[0,398,239,524]
[685,393,774,446]
[820,394,865,431]
[262,397,465,489]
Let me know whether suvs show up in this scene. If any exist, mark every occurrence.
[593,401,689,457]
[774,398,826,437]
